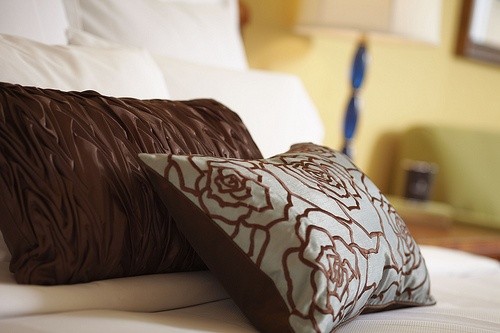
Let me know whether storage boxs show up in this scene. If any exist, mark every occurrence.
[0,80,267,286]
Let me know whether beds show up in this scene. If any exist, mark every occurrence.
[1,241,500,333]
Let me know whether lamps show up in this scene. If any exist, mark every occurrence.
[285,0,446,161]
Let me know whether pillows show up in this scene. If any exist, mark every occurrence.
[136,142,439,333]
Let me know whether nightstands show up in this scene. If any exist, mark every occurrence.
[384,194,499,265]
